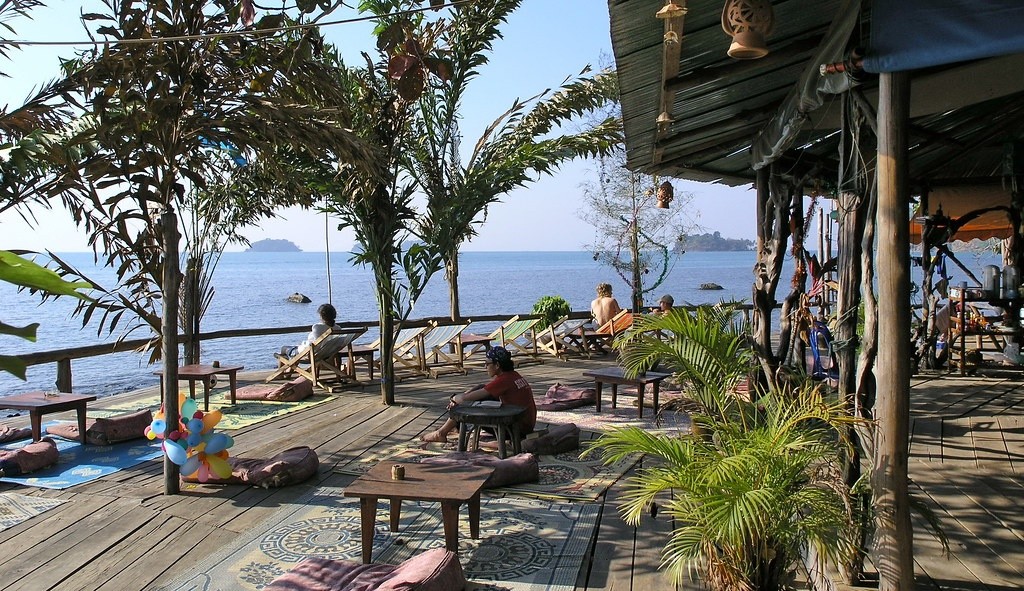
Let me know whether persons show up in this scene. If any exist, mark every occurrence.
[420,345,536,442]
[275,304,342,379]
[591,283,621,330]
[659,294,674,319]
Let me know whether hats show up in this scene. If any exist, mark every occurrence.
[486,346,511,361]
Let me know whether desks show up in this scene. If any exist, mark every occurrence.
[448,334,496,355]
[345,460,497,564]
[0,391,96,444]
[450,405,526,460]
[564,332,611,356]
[153,364,245,412]
[583,366,671,417]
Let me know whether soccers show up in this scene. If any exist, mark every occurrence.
[200,374,217,388]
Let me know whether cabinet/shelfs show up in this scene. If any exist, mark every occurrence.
[948,287,1024,376]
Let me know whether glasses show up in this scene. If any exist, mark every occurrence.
[660,301,665,305]
[485,360,495,366]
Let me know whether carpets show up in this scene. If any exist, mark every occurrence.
[0,384,751,591]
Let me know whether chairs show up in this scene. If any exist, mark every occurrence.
[265,307,652,393]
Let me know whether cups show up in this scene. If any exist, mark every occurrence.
[44,389,60,397]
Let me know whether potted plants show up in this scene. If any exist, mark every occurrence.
[532,296,571,350]
[672,301,751,448]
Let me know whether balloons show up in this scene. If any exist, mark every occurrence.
[144,394,234,482]
